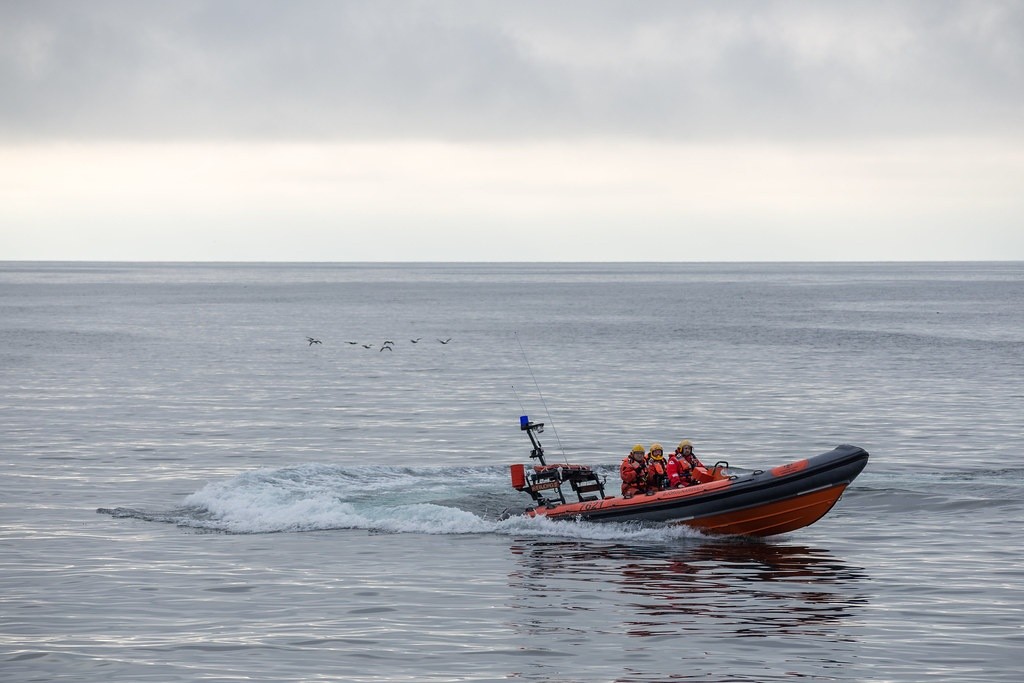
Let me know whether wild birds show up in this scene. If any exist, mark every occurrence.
[304,336,452,352]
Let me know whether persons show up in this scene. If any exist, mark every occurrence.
[620,440,703,497]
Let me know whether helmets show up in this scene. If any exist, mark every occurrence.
[679,439,693,452]
[650,443,663,460]
[632,444,645,456]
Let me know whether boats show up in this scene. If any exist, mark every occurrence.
[497,415,871,541]
